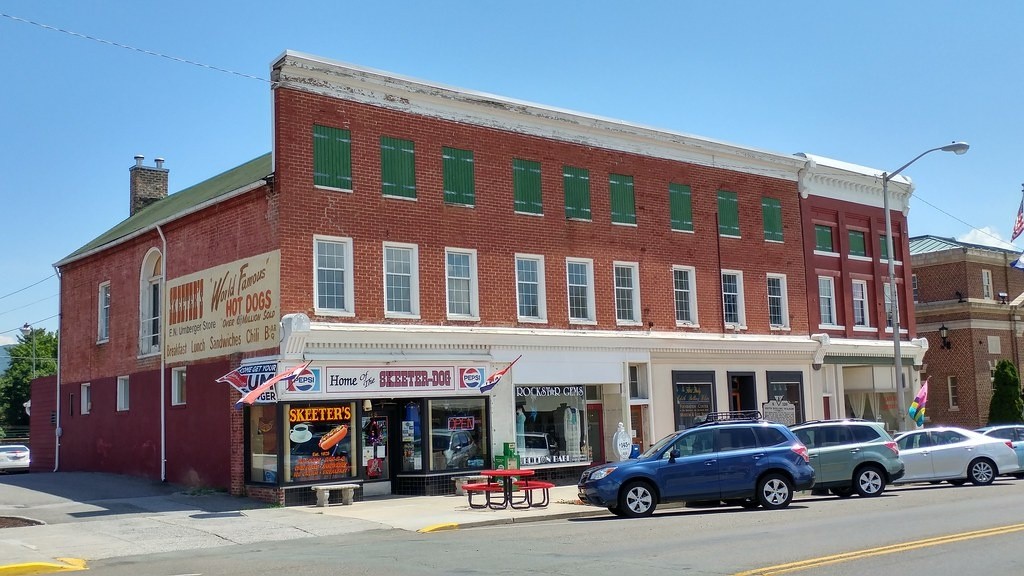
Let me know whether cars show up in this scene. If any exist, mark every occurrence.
[971,425,1024,479]
[0,445,30,471]
[516,431,559,465]
[892,427,1019,486]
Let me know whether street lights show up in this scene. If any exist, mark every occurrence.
[20,324,35,378]
[882,142,969,438]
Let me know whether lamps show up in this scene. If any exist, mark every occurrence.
[998,292,1008,304]
[939,323,951,349]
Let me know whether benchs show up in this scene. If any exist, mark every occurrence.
[451,475,492,496]
[513,481,555,507]
[311,483,360,507]
[462,483,504,509]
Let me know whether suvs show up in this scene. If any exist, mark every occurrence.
[410,430,476,470]
[788,418,903,498]
[578,410,815,518]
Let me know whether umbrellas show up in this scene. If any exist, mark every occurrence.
[1010,250,1024,270]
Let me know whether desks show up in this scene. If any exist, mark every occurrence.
[480,469,534,510]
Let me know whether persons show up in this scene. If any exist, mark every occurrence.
[516,406,526,453]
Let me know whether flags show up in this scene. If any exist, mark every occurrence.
[234,363,306,409]
[480,364,510,394]
[908,381,928,427]
[1011,192,1024,243]
